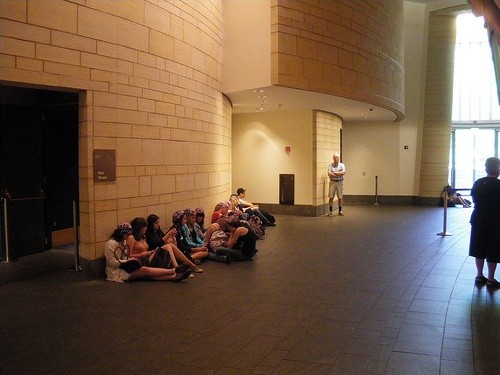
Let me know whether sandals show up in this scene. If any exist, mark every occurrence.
[486,279,500,288]
[475,275,487,284]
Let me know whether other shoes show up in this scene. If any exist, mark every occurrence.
[263,221,276,226]
[338,211,344,216]
[328,211,332,216]
[171,264,203,282]
[225,252,232,264]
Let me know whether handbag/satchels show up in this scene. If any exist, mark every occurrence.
[114,244,142,273]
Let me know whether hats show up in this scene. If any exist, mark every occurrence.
[116,222,132,234]
[172,210,184,222]
[184,208,196,216]
[237,188,246,193]
[195,208,204,214]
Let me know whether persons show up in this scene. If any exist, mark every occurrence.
[204,187,277,264]
[194,207,206,232]
[326,153,346,216]
[467,157,500,291]
[145,214,197,266]
[165,209,209,263]
[103,221,196,282]
[126,217,204,279]
[184,207,231,264]
[441,185,472,209]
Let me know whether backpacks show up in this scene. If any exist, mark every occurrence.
[260,209,275,223]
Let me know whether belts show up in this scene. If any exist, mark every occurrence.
[331,179,344,182]
